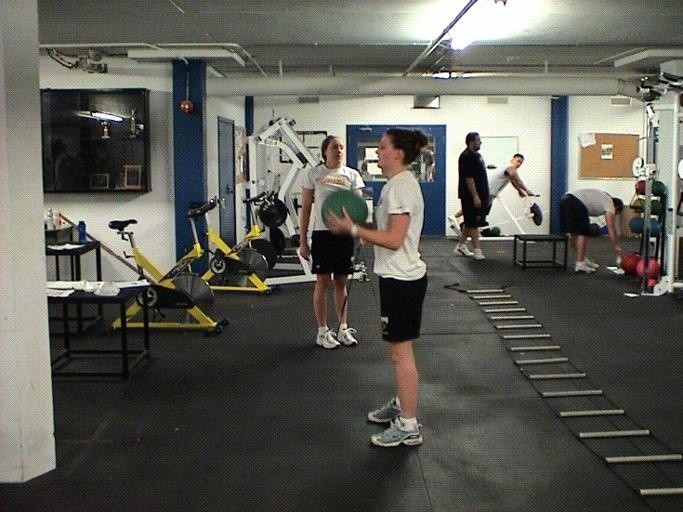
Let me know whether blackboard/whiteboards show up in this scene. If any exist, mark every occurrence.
[478,136,519,173]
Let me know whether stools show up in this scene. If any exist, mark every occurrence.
[511,234,568,271]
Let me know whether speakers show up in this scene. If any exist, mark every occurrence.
[298,97,320,102]
[611,98,630,105]
[488,97,508,104]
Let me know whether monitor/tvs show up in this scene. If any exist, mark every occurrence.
[40,88,152,194]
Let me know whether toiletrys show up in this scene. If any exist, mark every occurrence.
[78,221,86,242]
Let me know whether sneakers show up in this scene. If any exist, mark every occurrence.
[575,261,596,273]
[450,225,464,238]
[336,328,359,347]
[367,396,401,423]
[371,416,423,447]
[316,327,340,350]
[583,256,599,269]
[472,248,486,260]
[453,243,474,257]
[447,215,461,232]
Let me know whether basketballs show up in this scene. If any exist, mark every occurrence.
[623,253,660,278]
[491,227,501,235]
[636,181,665,196]
[321,190,368,231]
[632,199,663,214]
[629,217,661,237]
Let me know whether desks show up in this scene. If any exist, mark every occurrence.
[47,278,147,380]
[43,240,103,340]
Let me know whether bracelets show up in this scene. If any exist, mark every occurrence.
[350,223,359,240]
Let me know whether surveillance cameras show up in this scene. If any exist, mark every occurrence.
[88,49,103,61]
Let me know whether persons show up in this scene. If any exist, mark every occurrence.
[299,134,365,349]
[559,188,624,273]
[448,153,535,233]
[322,129,429,449]
[452,132,490,260]
